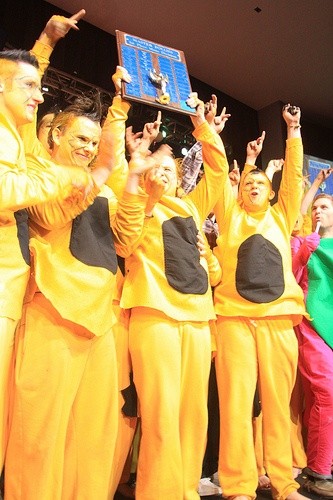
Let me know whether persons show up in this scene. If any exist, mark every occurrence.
[18,10,333,500]
[0,51,115,500]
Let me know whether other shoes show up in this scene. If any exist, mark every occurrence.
[300,474,333,498]
[253,473,273,492]
[115,481,135,498]
[197,477,223,497]
